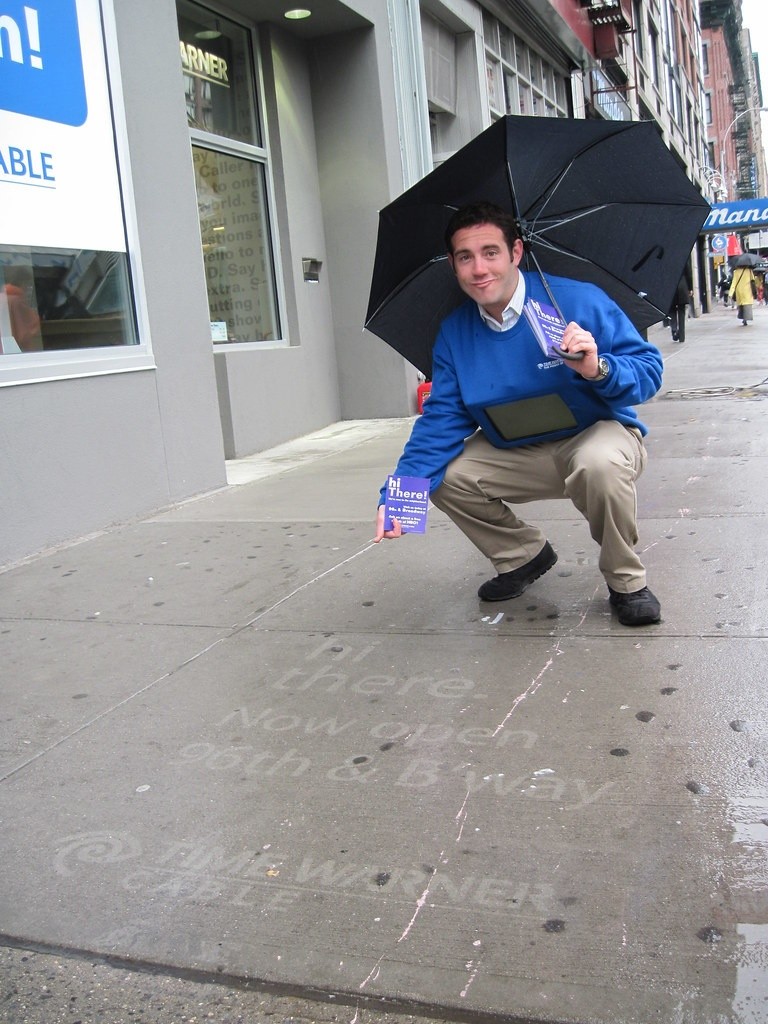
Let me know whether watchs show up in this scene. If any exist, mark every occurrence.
[581,358,609,382]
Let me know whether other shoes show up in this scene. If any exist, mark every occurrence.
[672,331,678,341]
[679,336,685,342]
[742,320,744,323]
[744,320,747,326]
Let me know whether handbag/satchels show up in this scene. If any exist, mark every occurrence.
[732,290,736,301]
[750,279,757,300]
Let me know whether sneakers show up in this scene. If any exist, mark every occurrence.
[477,540,559,601]
[606,584,662,626]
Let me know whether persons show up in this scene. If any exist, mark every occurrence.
[669,262,693,342]
[372,202,661,628]
[718,275,730,306]
[728,266,756,326]
[0,268,41,355]
[754,264,768,306]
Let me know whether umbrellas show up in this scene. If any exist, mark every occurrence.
[361,114,712,381]
[727,253,764,266]
